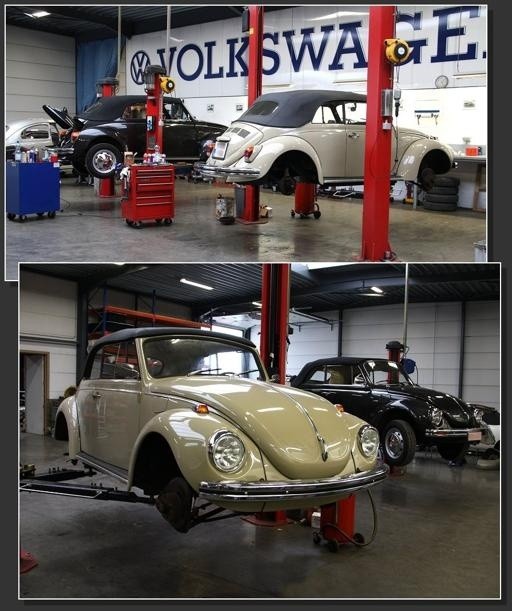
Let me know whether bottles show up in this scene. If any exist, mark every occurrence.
[14,141,22,164]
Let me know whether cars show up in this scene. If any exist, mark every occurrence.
[5,95,232,182]
[193,86,455,193]
[290,357,496,464]
[51,326,393,534]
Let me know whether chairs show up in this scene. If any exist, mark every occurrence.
[328,369,351,384]
